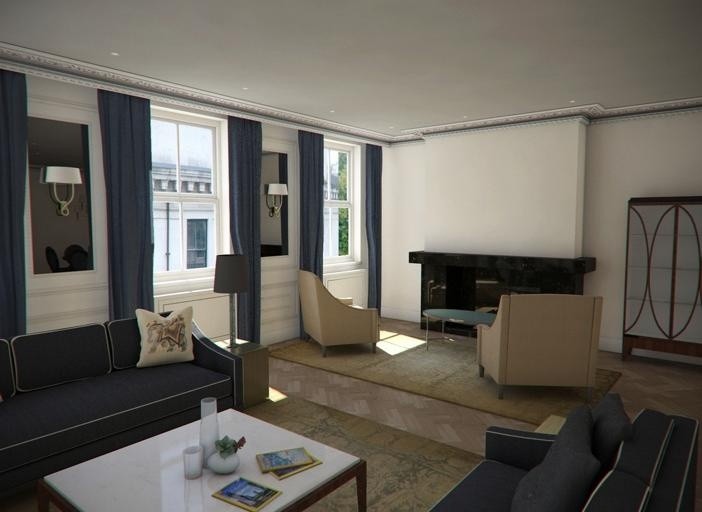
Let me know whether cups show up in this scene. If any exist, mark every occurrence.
[198,395,221,470]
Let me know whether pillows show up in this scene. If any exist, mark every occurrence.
[131,305,198,370]
[586,388,647,470]
[507,400,603,512]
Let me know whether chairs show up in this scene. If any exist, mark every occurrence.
[294,267,381,358]
[471,291,607,405]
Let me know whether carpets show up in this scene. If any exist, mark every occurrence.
[0,387,487,512]
[261,317,628,430]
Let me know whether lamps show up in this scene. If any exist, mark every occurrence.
[42,165,84,216]
[205,253,271,408]
[265,181,289,218]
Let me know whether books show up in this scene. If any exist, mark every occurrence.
[255,445,315,473]
[211,474,284,512]
[270,453,323,481]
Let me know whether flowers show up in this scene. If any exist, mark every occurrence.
[213,432,248,460]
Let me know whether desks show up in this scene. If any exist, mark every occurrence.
[422,305,498,366]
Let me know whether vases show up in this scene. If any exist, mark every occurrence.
[206,450,241,475]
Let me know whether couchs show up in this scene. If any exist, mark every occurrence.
[424,388,698,512]
[1,309,246,503]
